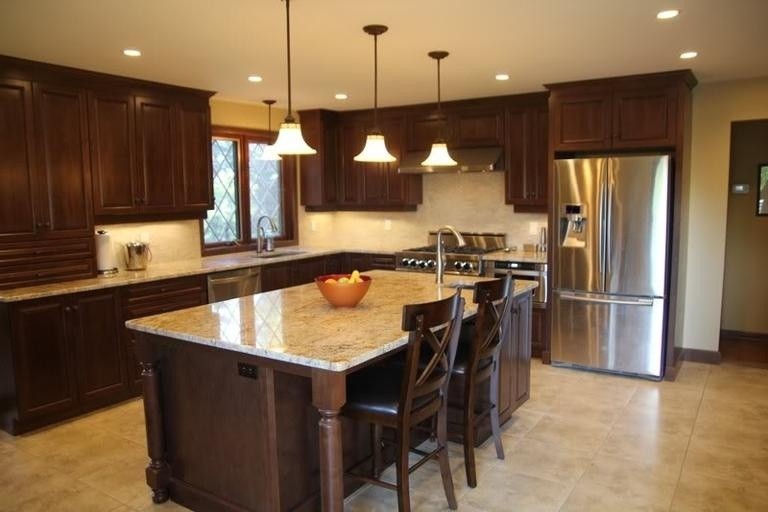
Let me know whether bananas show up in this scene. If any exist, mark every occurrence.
[350,270,359,283]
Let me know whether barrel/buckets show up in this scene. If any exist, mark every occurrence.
[124,241,152,270]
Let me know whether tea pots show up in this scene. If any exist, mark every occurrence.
[119,239,151,271]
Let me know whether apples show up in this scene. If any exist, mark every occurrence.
[325,277,364,283]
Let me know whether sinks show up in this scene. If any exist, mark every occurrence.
[445,282,475,290]
[250,251,307,259]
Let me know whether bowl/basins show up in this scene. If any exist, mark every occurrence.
[313,273,371,308]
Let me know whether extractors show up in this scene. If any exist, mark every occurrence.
[396,146,506,174]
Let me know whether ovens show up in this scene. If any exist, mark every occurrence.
[482,259,547,310]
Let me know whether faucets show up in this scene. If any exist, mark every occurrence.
[436,225,465,284]
[257,216,277,253]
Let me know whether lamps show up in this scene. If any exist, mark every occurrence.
[421,52,458,166]
[353,24,399,163]
[273,0,318,156]
[255,100,284,160]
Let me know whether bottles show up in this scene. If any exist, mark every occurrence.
[540,227,547,253]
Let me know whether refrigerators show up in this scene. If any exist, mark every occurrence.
[550,152,672,383]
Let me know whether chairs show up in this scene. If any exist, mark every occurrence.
[340,288,465,512]
[418,271,515,488]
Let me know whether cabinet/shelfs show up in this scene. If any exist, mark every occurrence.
[297,94,546,212]
[0,62,96,279]
[122,277,206,410]
[263,254,395,291]
[0,288,121,439]
[554,88,676,151]
[87,72,211,223]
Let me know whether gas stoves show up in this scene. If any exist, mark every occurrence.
[395,243,499,276]
